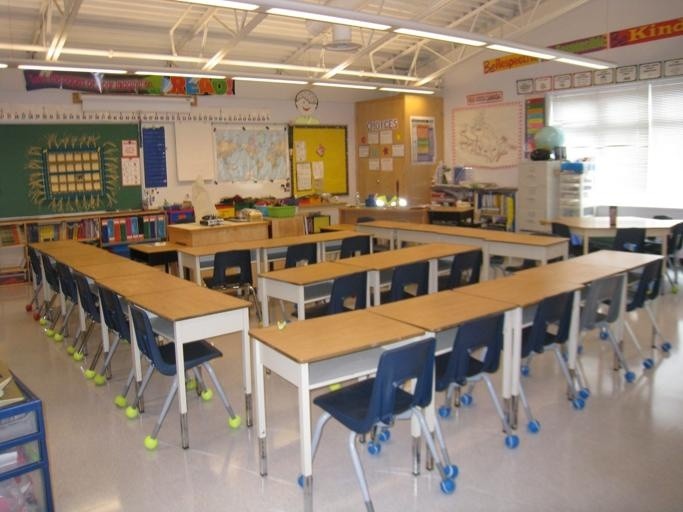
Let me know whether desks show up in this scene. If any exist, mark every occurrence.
[338,204,429,224]
[429,206,474,224]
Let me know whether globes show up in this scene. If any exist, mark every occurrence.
[533,126,564,160]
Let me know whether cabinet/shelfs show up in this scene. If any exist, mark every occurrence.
[0,362,54,512]
[0,216,33,282]
[559,172,597,219]
[516,160,570,236]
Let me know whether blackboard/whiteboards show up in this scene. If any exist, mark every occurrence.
[292,125,349,199]
[141,120,292,211]
[0,123,143,222]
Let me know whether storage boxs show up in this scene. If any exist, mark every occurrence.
[254,205,269,216]
[215,204,236,218]
[267,205,296,219]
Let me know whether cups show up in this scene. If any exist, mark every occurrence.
[609,206,617,226]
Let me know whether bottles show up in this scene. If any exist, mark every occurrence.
[554,146,561,160]
[561,146,567,160]
[355,192,361,208]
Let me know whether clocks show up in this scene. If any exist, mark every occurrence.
[295,90,319,113]
